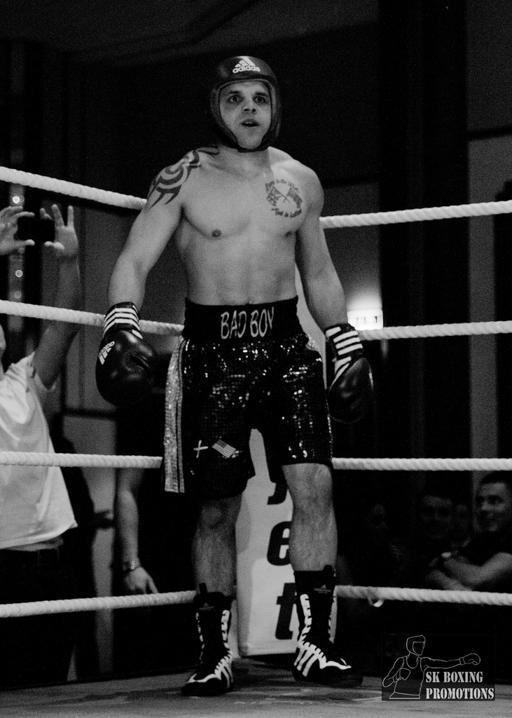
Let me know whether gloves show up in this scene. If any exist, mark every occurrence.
[323,323,375,426]
[96,303,157,408]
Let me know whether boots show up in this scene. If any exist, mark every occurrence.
[291,570,362,688]
[182,588,238,698]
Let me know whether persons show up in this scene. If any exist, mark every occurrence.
[0,204,512,692]
[97,56,374,696]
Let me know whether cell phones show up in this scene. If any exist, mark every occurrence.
[13,215,56,244]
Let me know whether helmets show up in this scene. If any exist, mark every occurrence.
[208,55,280,148]
